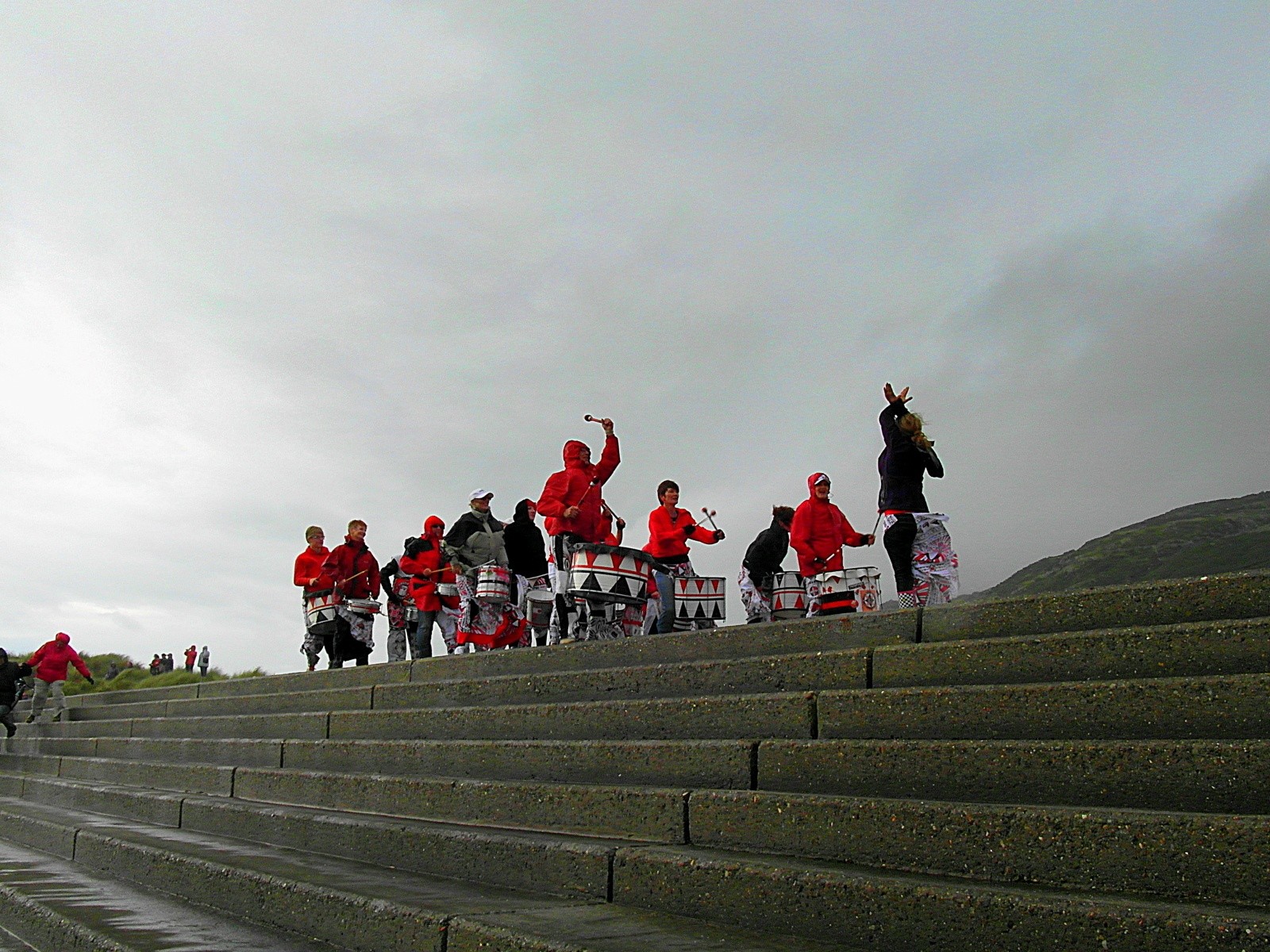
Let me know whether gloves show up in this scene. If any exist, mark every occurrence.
[86,676,94,685]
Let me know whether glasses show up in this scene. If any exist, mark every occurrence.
[432,525,444,528]
[310,535,325,540]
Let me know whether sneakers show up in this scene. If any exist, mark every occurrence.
[26,715,35,723]
[52,712,61,721]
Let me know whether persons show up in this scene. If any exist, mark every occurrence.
[294,418,725,672]
[739,505,795,624]
[18,633,94,723]
[149,645,210,676]
[0,648,32,738]
[878,383,944,610]
[103,660,137,682]
[790,473,877,619]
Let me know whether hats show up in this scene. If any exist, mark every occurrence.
[468,488,494,501]
[305,526,323,544]
[814,474,830,485]
[527,500,537,512]
[55,632,70,645]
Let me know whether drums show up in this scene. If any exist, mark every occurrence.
[673,576,727,620]
[565,542,653,604]
[347,599,383,614]
[435,582,460,597]
[403,606,420,623]
[771,571,809,618]
[475,565,510,603]
[525,589,554,627]
[802,566,884,616]
[301,589,339,635]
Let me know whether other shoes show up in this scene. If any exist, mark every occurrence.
[7,724,16,737]
